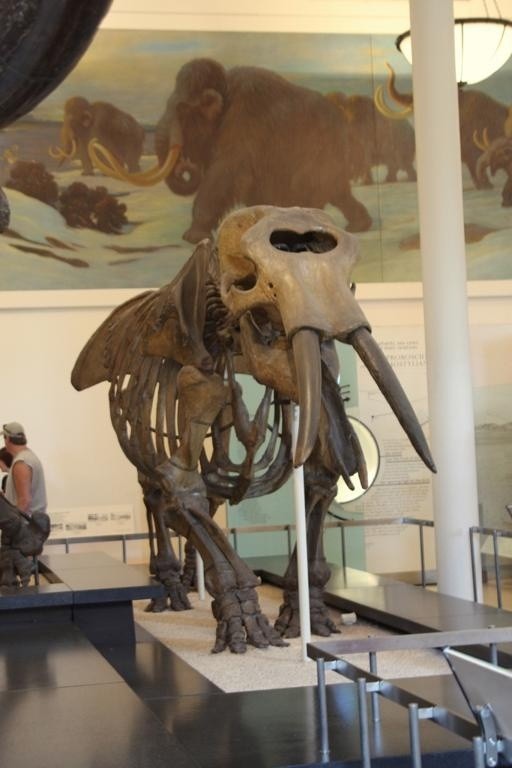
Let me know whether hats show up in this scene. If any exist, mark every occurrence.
[0,421,25,438]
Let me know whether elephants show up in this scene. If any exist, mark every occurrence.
[48,57,510,246]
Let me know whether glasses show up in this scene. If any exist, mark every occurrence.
[2,424,14,435]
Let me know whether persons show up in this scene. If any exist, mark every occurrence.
[1,446,14,496]
[1,421,48,523]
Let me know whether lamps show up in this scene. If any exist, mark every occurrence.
[395,0,512,89]
